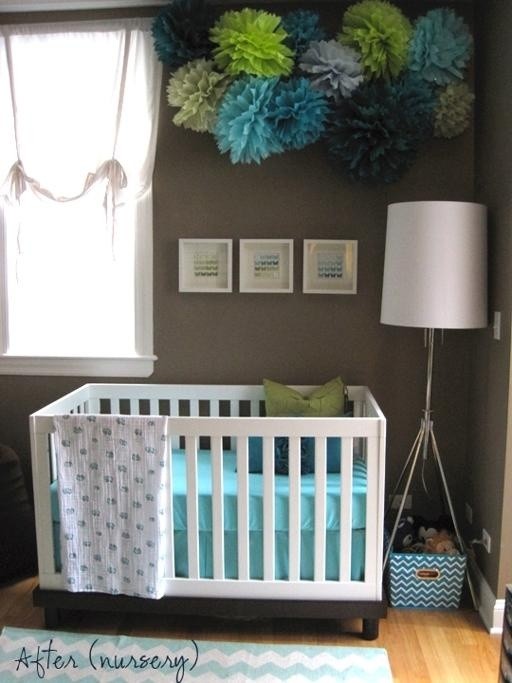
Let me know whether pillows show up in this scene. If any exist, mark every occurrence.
[249,376,346,477]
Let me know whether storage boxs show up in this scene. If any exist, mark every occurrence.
[387,538,468,611]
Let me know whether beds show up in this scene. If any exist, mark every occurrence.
[28,380,389,641]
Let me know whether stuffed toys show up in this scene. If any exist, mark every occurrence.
[390,516,455,554]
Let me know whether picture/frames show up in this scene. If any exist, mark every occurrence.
[179,237,359,295]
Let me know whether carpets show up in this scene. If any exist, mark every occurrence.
[1,623,394,683]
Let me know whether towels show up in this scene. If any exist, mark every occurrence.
[52,413,171,602]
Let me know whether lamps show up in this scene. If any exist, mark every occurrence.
[380,199,491,612]
